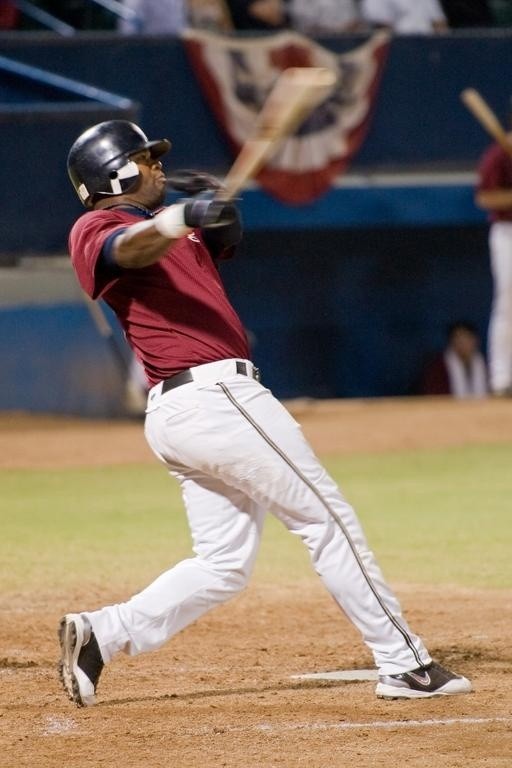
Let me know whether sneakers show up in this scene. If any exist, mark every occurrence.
[370,659,475,702]
[55,610,105,709]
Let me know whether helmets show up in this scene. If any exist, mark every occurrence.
[61,119,173,209]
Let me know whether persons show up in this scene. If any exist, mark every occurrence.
[58,118,479,711]
[419,320,488,397]
[1,0,454,39]
[475,109,511,400]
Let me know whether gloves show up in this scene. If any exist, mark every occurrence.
[160,163,224,193]
[168,195,242,231]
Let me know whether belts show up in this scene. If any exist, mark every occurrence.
[142,357,262,399]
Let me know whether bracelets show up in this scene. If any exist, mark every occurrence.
[152,202,197,241]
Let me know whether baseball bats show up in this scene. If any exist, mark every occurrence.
[461,90,511,157]
[220,69,328,200]
